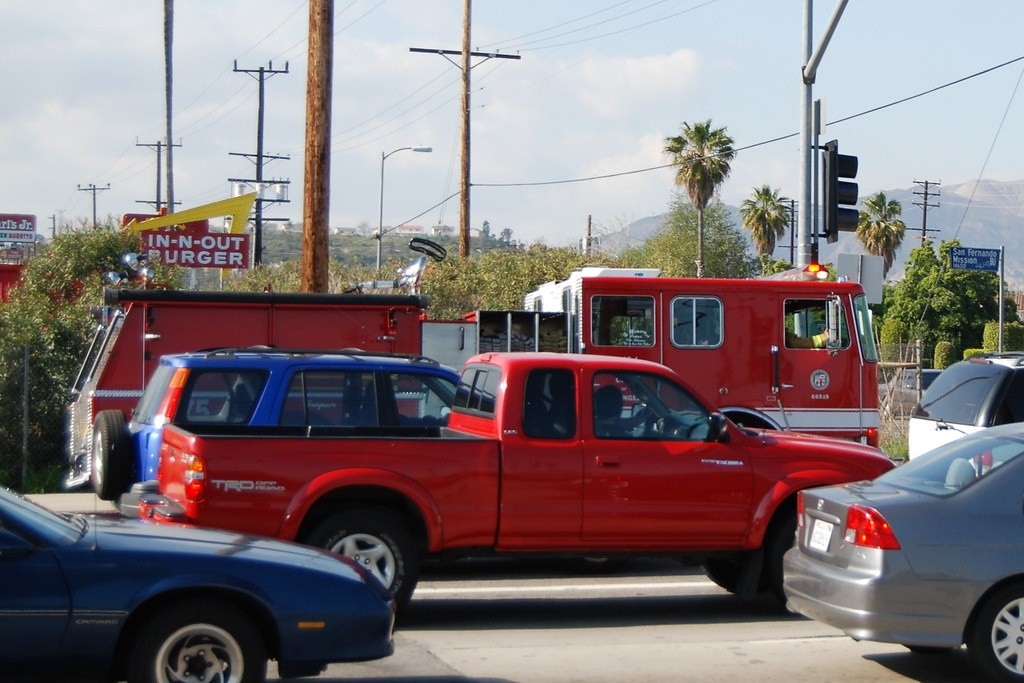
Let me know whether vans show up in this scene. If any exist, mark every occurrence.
[891,369,945,422]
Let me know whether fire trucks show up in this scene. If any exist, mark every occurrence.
[63,255,882,490]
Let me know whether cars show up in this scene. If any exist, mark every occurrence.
[0,487,398,683]
[782,423,1023,683]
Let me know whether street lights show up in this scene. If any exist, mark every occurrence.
[374,144,432,277]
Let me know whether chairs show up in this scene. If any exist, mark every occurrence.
[346,373,362,416]
[946,458,977,487]
[232,385,252,422]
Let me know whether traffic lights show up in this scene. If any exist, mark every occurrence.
[820,137,861,244]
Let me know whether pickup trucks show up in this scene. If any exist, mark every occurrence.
[118,350,900,625]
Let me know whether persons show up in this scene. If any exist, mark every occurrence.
[784,328,826,349]
[366,381,449,426]
[546,369,654,440]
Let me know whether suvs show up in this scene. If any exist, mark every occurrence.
[907,351,1023,479]
[91,342,496,516]
